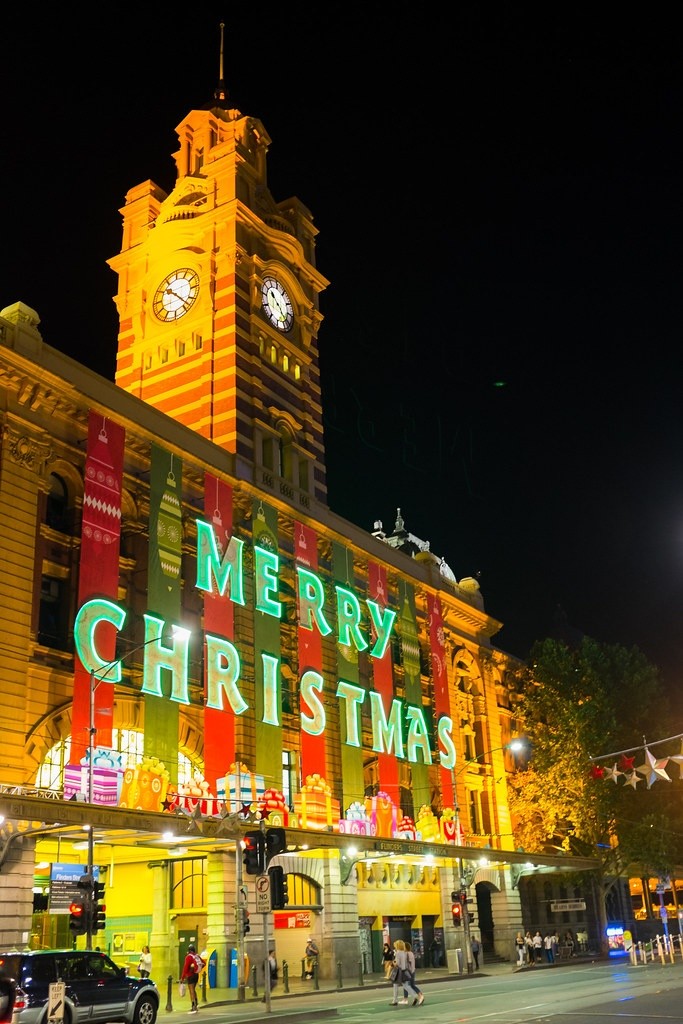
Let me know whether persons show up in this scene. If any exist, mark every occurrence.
[514,927,589,966]
[139,946,151,978]
[90,947,105,973]
[180,944,206,1014]
[469,936,479,970]
[381,943,394,979]
[261,950,279,1003]
[429,937,439,968]
[304,940,318,979]
[389,941,417,1006]
[398,942,424,1006]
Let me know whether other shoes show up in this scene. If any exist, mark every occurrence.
[398,999,408,1005]
[188,998,198,1014]
[412,994,424,1005]
[305,974,313,979]
[389,1002,397,1005]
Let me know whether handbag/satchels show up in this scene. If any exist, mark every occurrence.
[389,965,399,982]
[137,963,141,972]
[180,982,186,996]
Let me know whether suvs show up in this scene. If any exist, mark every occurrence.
[0,950,160,1024]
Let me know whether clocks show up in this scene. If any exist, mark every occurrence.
[152,267,200,322]
[261,278,294,334]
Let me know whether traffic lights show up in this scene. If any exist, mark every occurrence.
[78,875,95,895]
[69,897,87,936]
[451,891,461,903]
[266,828,286,852]
[244,911,250,937]
[453,904,461,926]
[243,831,264,875]
[91,881,107,935]
[268,866,289,910]
[466,899,475,923]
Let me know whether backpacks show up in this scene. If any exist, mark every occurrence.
[188,953,205,974]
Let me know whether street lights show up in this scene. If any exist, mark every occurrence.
[451,742,523,975]
[86,631,187,951]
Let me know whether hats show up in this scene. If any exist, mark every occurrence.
[307,939,311,942]
[189,944,196,949]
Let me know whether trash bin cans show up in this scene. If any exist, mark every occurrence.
[446,949,464,974]
